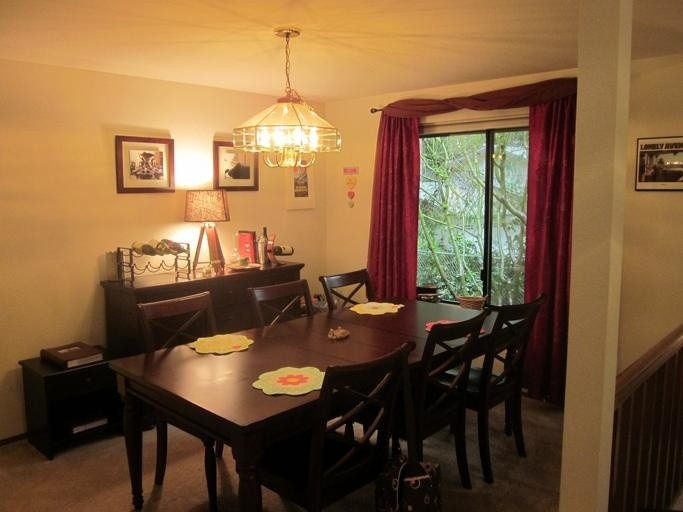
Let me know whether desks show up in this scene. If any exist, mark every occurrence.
[108,297,526,512]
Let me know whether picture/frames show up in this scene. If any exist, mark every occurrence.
[115,136,175,193]
[635,136,683,191]
[213,140,259,191]
[285,164,316,210]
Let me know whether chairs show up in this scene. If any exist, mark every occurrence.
[254,341,416,512]
[135,291,224,512]
[246,278,314,326]
[436,293,547,483]
[319,268,375,311]
[342,307,491,490]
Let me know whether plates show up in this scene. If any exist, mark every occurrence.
[227,262,259,271]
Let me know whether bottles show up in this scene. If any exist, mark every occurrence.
[258,226,271,266]
[132,238,192,258]
[268,246,293,256]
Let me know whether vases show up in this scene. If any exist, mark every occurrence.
[459,296,485,310]
[416,285,440,303]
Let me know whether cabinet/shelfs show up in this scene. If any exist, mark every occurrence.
[18,345,124,460]
[101,258,304,433]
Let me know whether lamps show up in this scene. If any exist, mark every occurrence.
[233,27,342,169]
[184,190,230,274]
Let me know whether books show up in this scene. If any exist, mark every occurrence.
[53,401,110,435]
[40,340,103,371]
[237,230,256,264]
